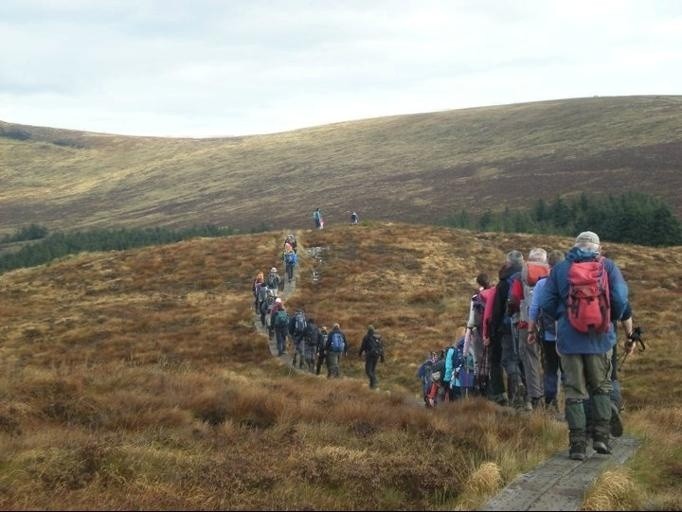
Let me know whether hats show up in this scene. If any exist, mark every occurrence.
[270,268,277,272]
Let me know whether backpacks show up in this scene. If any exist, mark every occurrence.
[503,271,523,325]
[520,262,549,324]
[330,333,346,352]
[294,315,305,330]
[277,313,289,328]
[567,256,610,333]
[318,335,327,347]
[369,334,382,355]
[308,328,318,346]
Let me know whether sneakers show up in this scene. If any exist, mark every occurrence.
[570,444,587,459]
[609,405,624,437]
[592,441,612,454]
[522,399,561,415]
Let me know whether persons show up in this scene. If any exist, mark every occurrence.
[417,249,626,437]
[282,233,298,282]
[252,268,386,389]
[540,230,640,458]
[351,212,359,225]
[312,208,321,229]
